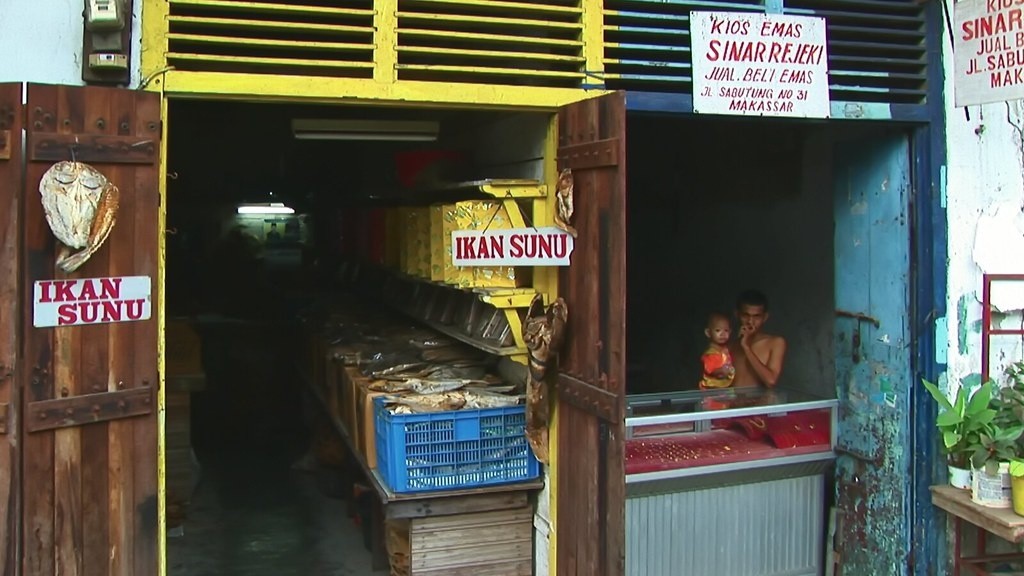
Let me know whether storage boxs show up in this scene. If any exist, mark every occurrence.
[290,309,540,495]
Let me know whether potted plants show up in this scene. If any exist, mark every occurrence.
[924,357,1023,516]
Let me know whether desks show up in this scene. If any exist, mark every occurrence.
[929,482,1024,576]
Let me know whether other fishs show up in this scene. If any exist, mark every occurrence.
[328,314,530,485]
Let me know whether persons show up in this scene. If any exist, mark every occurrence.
[698,308,736,411]
[727,291,788,391]
